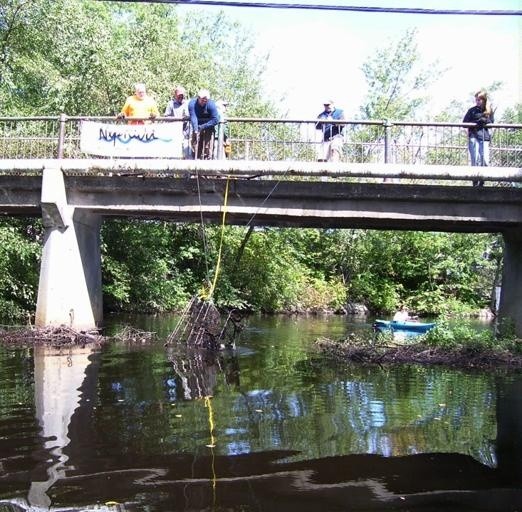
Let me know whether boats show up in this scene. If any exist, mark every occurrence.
[373,317,438,333]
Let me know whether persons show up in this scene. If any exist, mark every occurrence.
[462,90,495,187]
[314,98,345,161]
[116,83,230,161]
[392,305,408,322]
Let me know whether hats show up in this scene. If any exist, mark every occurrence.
[324,100,333,105]
[174,88,184,97]
[198,90,210,101]
